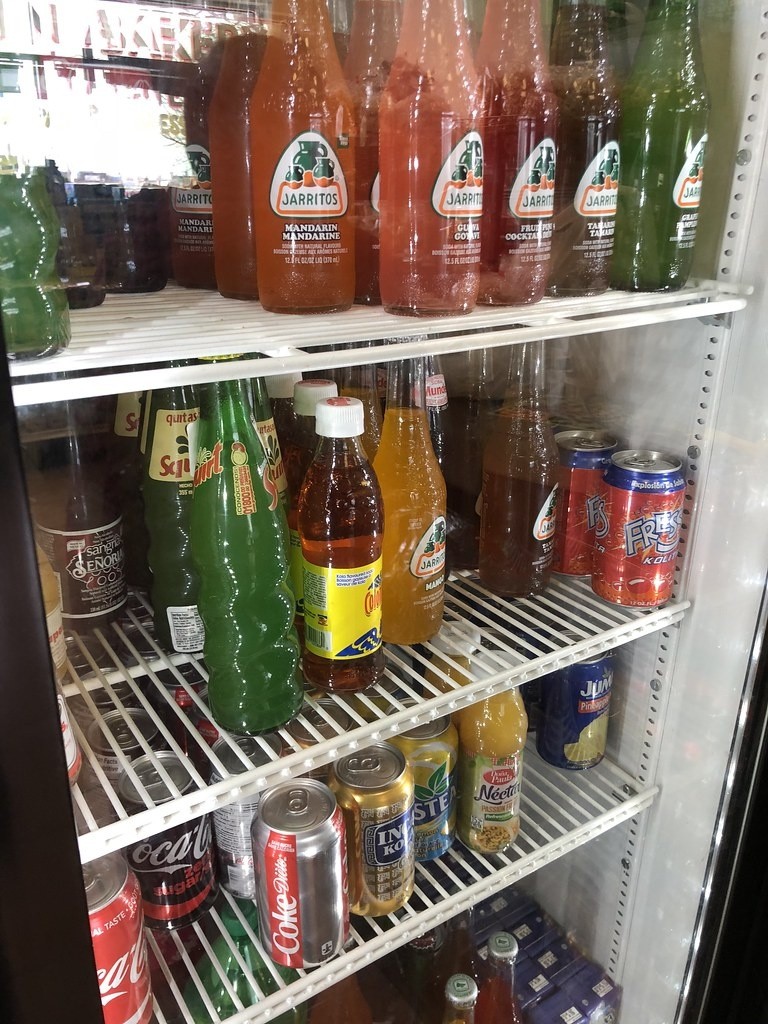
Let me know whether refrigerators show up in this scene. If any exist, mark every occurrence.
[0,0,768,1024]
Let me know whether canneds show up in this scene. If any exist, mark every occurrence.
[62,585,615,1024]
[552,430,619,580]
[590,449,688,610]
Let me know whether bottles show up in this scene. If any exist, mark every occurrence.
[422,619,530,853]
[151,880,523,1024]
[13,338,557,779]
[0,0,710,366]
